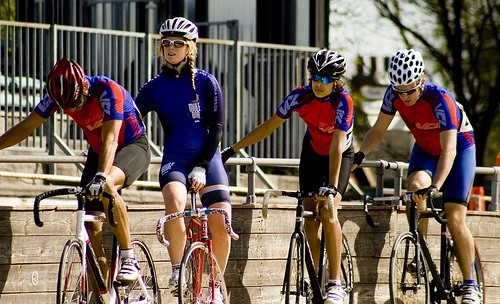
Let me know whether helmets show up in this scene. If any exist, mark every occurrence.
[388,49,424,86]
[307,50,346,79]
[159,17,198,40]
[46,58,85,111]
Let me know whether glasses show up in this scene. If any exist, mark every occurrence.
[70,94,82,108]
[161,39,188,48]
[311,74,335,84]
[392,78,422,95]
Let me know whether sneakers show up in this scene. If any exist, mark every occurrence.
[461,284,477,304]
[168,264,181,292]
[116,248,141,280]
[300,280,310,297]
[324,279,347,304]
[408,262,417,278]
[208,281,224,304]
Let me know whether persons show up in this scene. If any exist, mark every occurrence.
[0,55,150,304]
[351,49,479,304]
[134,16,232,304]
[220,48,355,304]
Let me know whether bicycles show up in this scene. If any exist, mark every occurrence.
[152,183,239,304]
[361,194,486,304]
[260,190,357,304]
[33,185,162,304]
